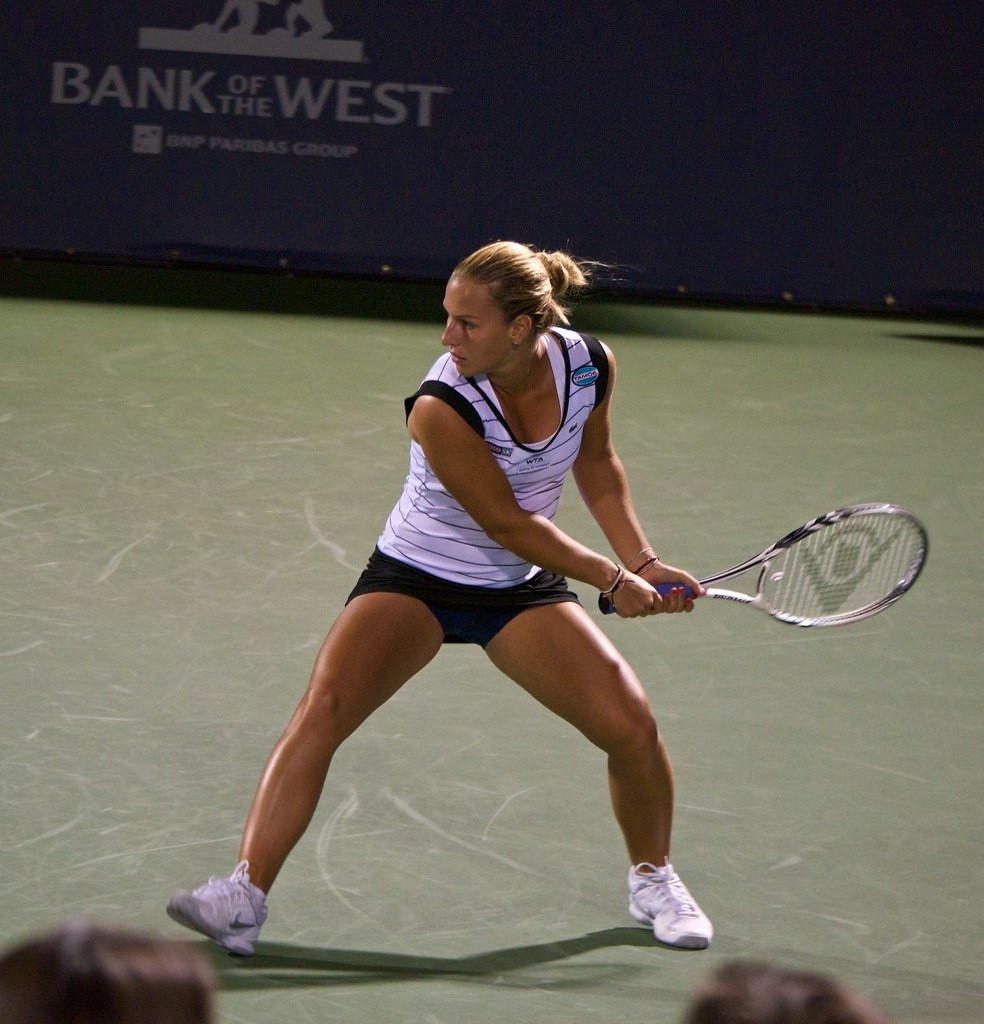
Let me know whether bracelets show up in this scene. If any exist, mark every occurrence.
[626,548,659,576]
[602,562,636,607]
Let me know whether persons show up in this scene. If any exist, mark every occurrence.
[165,241,714,959]
[1,930,210,1024]
[683,958,870,1024]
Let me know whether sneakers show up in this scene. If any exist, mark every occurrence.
[167,860,268,956]
[626,863,712,951]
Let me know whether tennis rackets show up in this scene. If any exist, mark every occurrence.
[596,501,931,629]
